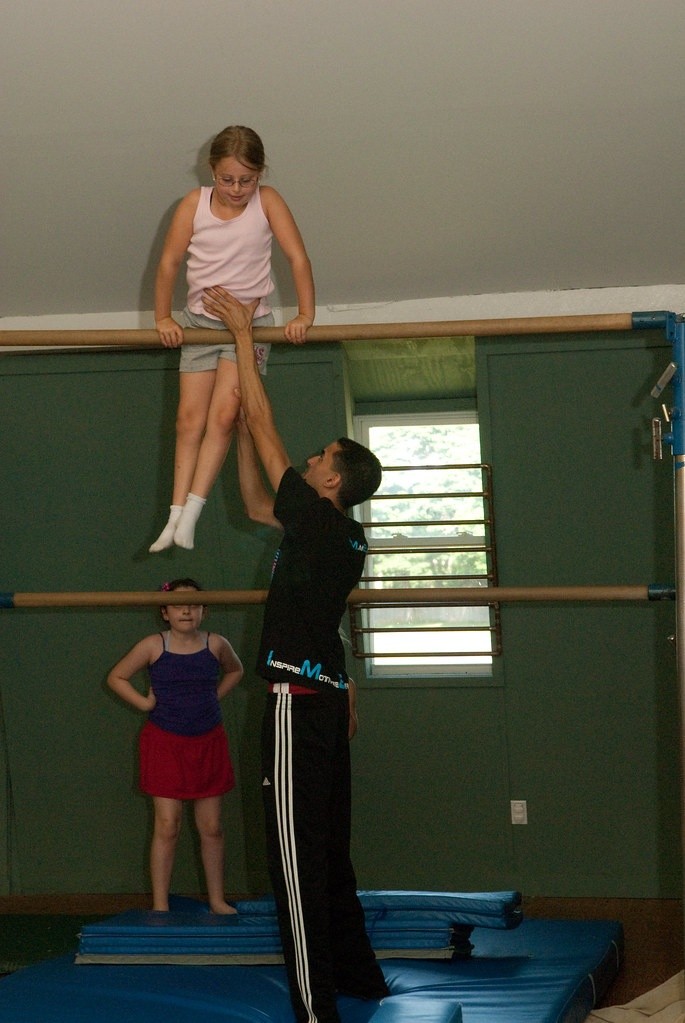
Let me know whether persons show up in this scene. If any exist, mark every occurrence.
[200,285,389,1022]
[108,581,243,914]
[148,125,316,552]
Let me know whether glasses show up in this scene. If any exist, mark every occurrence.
[209,166,260,188]
